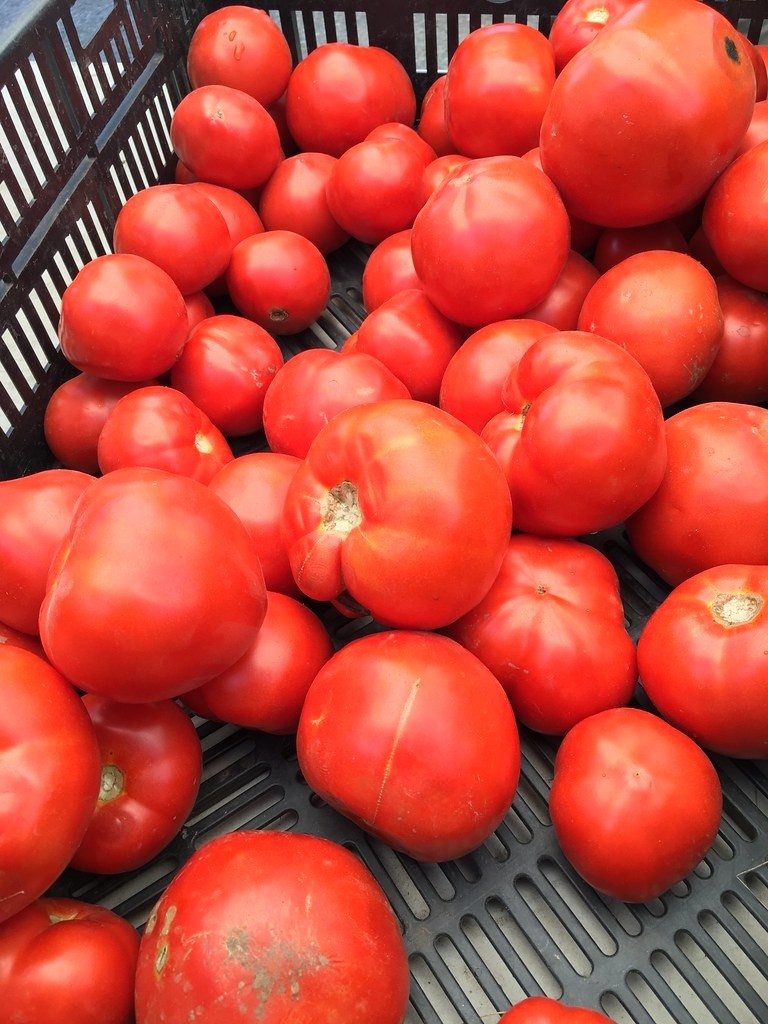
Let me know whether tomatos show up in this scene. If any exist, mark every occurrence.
[0,0,768,1024]
